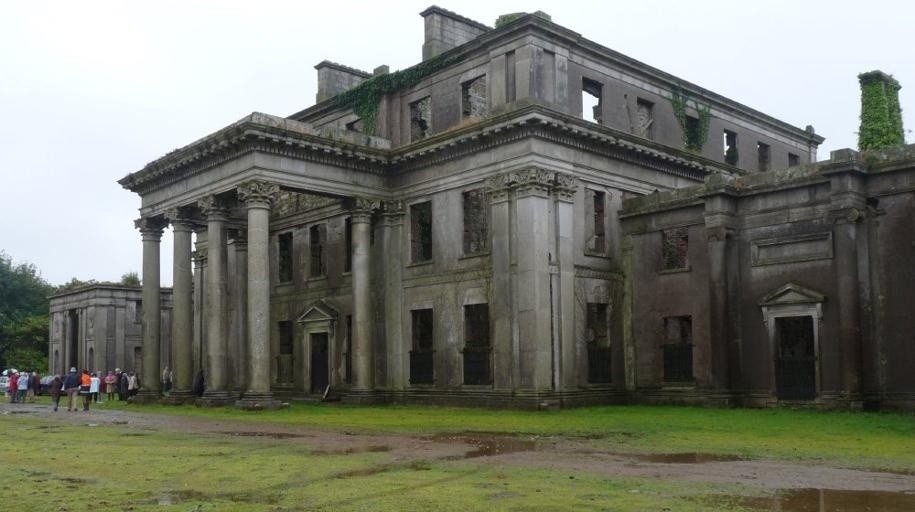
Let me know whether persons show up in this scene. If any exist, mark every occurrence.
[3,367,203,411]
